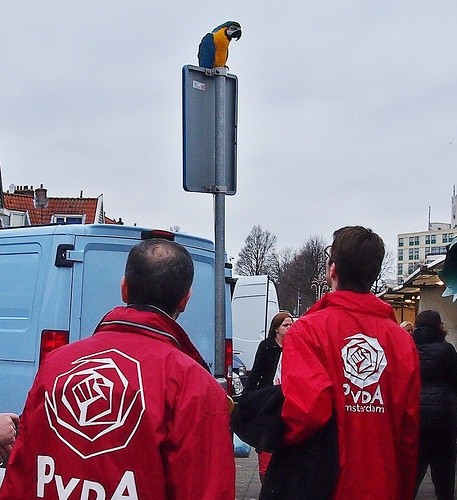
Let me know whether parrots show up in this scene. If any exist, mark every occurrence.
[197,19,243,70]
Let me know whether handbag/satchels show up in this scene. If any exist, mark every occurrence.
[233,433,250,458]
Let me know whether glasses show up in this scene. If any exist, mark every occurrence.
[323,245,333,264]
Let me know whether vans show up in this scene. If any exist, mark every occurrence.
[0,222,279,458]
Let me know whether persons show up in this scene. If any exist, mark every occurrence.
[266,224,422,500]
[0,412,21,464]
[0,237,239,500]
[398,320,415,336]
[410,309,457,500]
[232,311,296,500]
